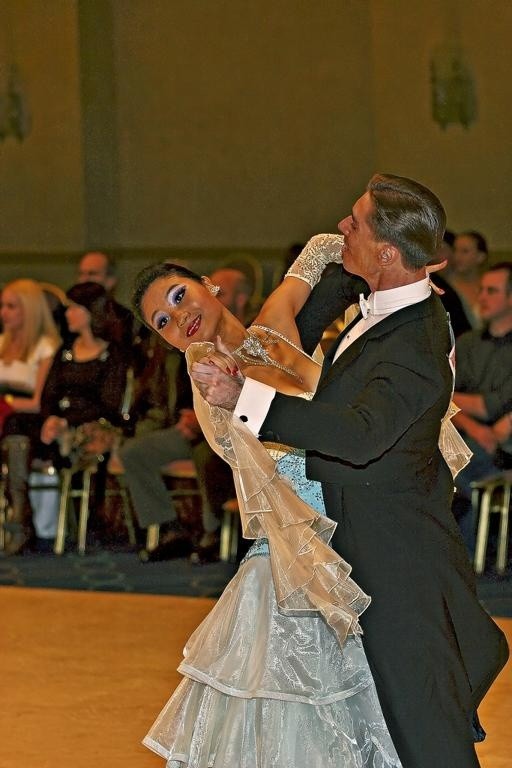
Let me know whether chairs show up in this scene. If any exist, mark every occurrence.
[0,251,510,578]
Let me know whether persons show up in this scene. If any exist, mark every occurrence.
[0,224,512,581]
[188,172,509,768]
[126,232,478,768]
